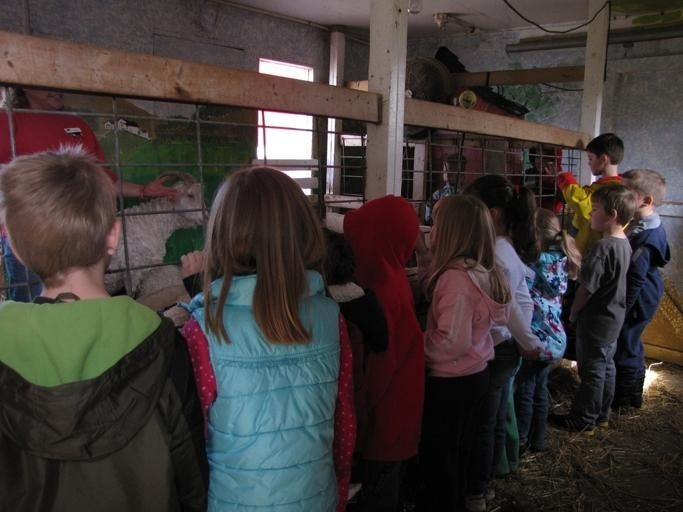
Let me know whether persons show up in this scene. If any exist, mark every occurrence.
[0,89,180,303]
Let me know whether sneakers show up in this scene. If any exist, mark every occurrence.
[464,488,495,512]
[547,410,608,435]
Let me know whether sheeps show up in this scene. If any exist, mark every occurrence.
[102,169,212,297]
